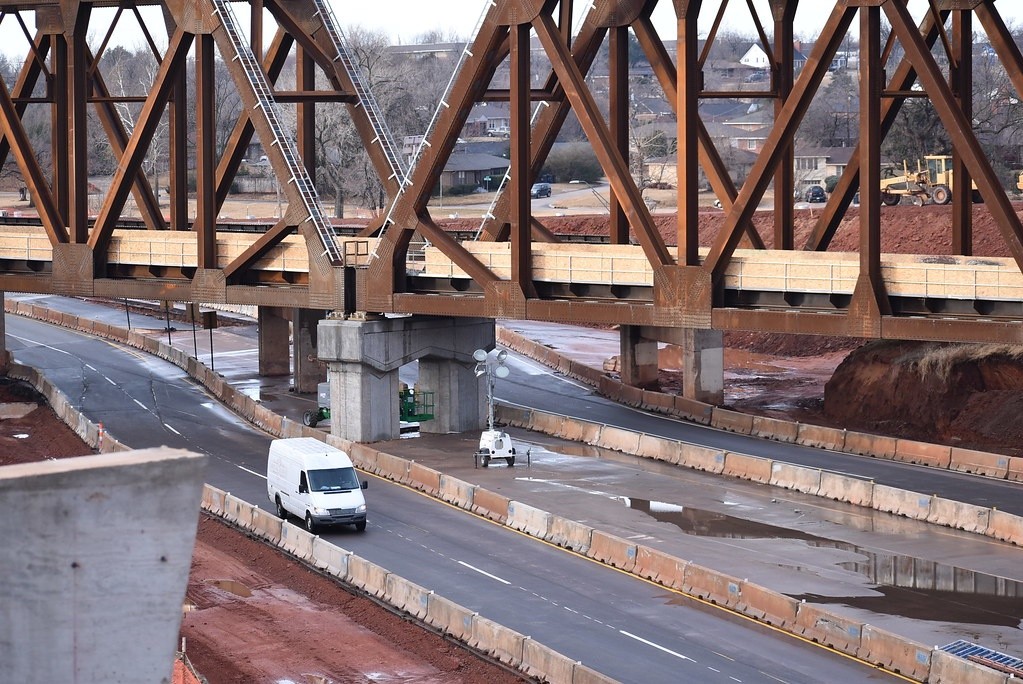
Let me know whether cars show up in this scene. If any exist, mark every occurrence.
[805,186,826,203]
[827,66,837,72]
[486,127,510,138]
[713,200,722,208]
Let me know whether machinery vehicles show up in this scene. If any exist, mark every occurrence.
[856,155,984,206]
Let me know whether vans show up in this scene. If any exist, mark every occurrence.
[266,437,370,532]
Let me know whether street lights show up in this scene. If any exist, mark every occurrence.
[472,349,509,431]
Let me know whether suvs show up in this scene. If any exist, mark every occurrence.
[531,183,551,198]
[744,74,764,83]
